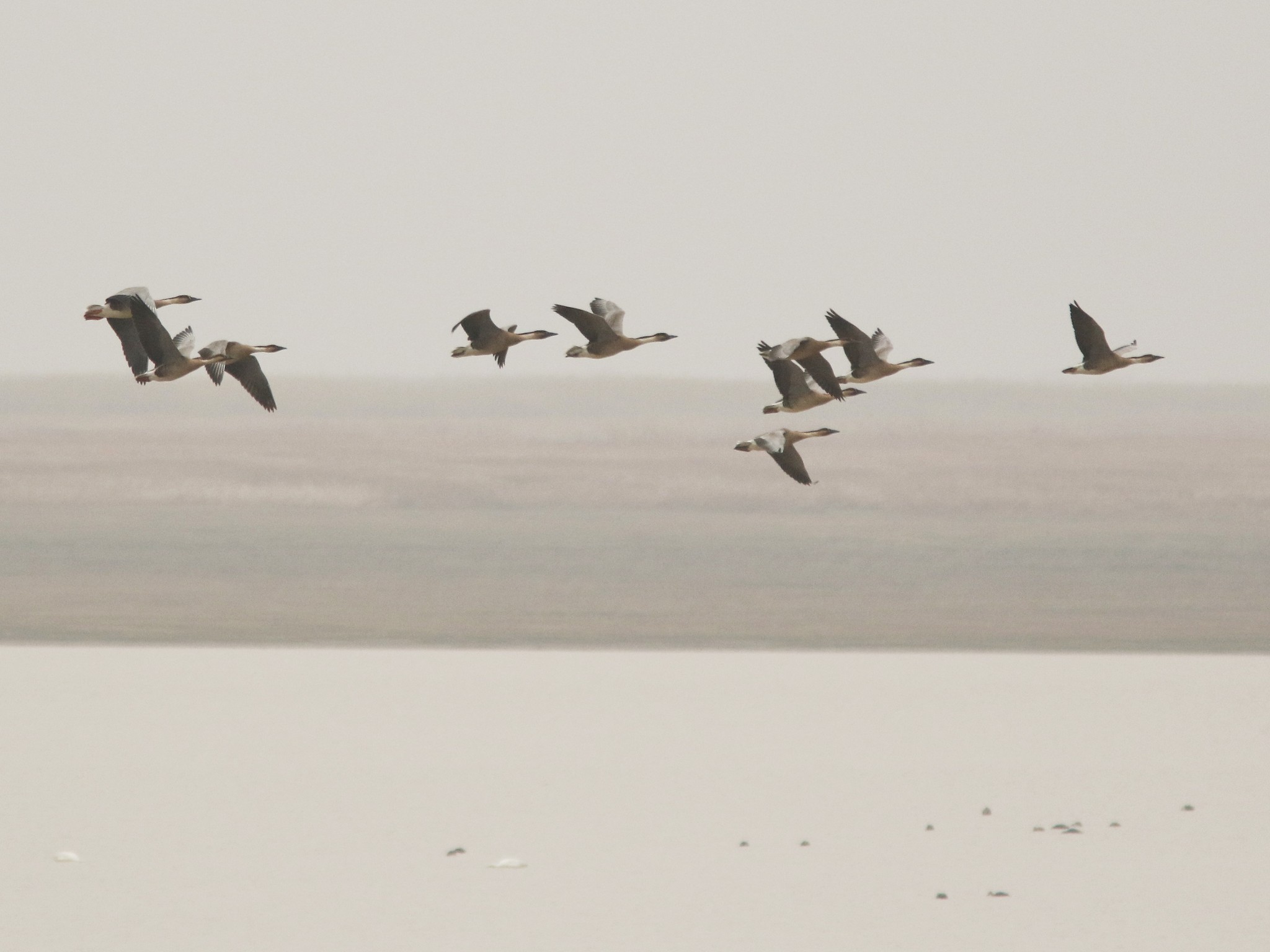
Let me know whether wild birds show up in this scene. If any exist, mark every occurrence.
[84,286,288,413]
[553,298,678,359]
[1061,300,1166,377]
[733,309,936,487]
[450,309,559,369]
